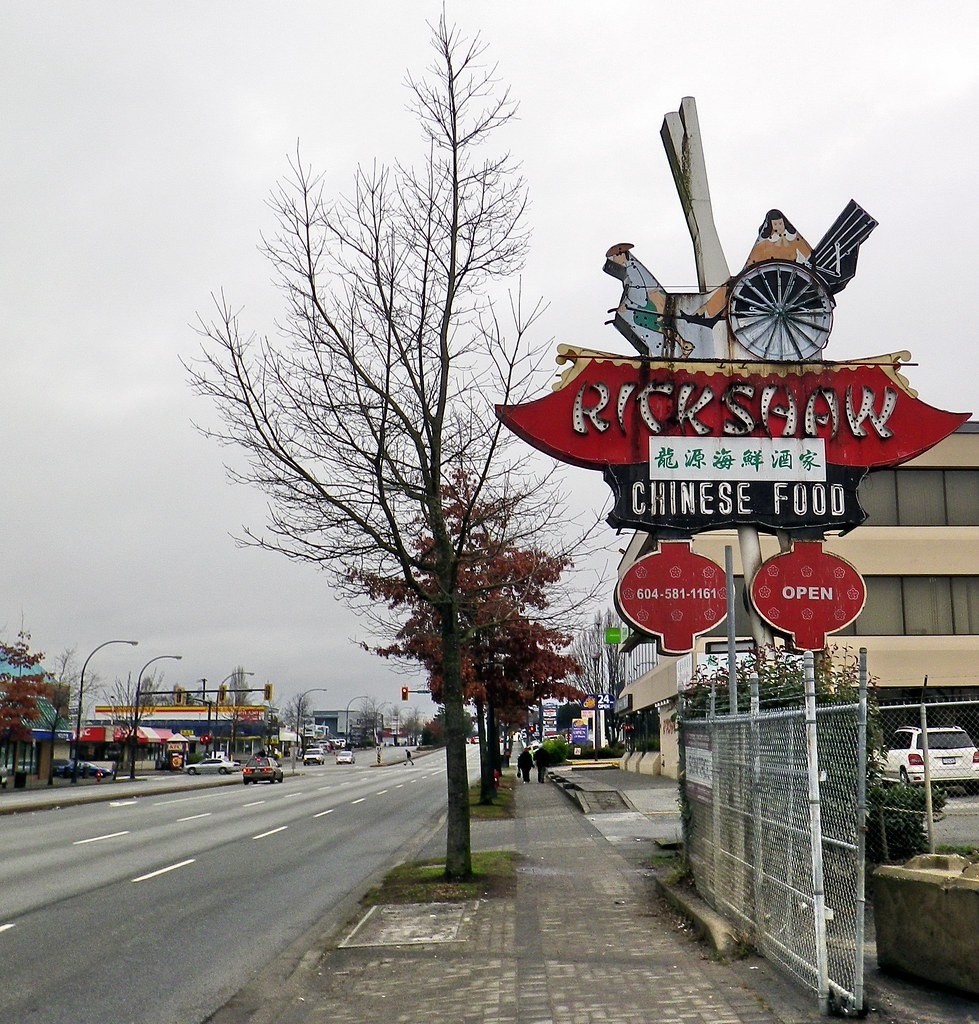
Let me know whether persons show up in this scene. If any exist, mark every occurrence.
[533,744,551,783]
[404,749,414,766]
[273,747,278,761]
[518,748,535,783]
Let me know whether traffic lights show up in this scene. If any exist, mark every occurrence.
[530,725,535,733]
[205,734,210,743]
[200,737,205,745]
[401,686,409,701]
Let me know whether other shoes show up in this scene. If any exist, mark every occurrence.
[539,781,541,782]
[542,781,544,783]
[412,763,414,765]
[524,779,530,782]
[404,764,407,766]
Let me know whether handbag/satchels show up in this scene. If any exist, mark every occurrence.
[517,771,522,778]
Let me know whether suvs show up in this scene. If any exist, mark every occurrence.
[872,725,979,797]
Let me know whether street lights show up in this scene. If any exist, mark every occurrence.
[214,672,255,757]
[372,702,393,741]
[295,688,328,761]
[345,696,368,745]
[70,640,139,784]
[130,654,183,776]
[395,707,412,746]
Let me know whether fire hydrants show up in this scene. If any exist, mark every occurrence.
[493,769,501,791]
[95,769,104,783]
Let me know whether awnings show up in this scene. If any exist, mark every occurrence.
[1,695,74,740]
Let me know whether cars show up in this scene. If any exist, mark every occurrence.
[241,755,284,785]
[335,751,356,765]
[15,757,69,778]
[303,747,326,766]
[62,760,112,779]
[306,738,350,753]
[465,735,480,744]
[183,757,241,776]
[499,729,526,743]
